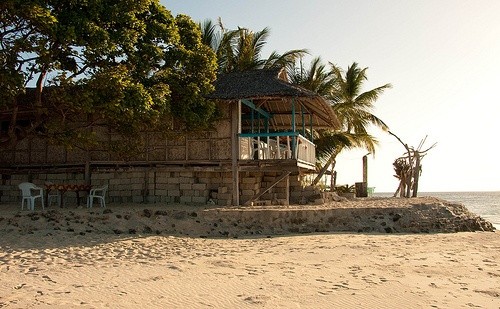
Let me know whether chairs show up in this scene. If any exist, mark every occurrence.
[86,184,108,208]
[48,189,61,208]
[18,182,44,210]
[250,139,292,159]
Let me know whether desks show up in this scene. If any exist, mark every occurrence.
[43,183,93,208]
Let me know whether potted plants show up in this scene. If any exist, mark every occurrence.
[338,184,357,198]
